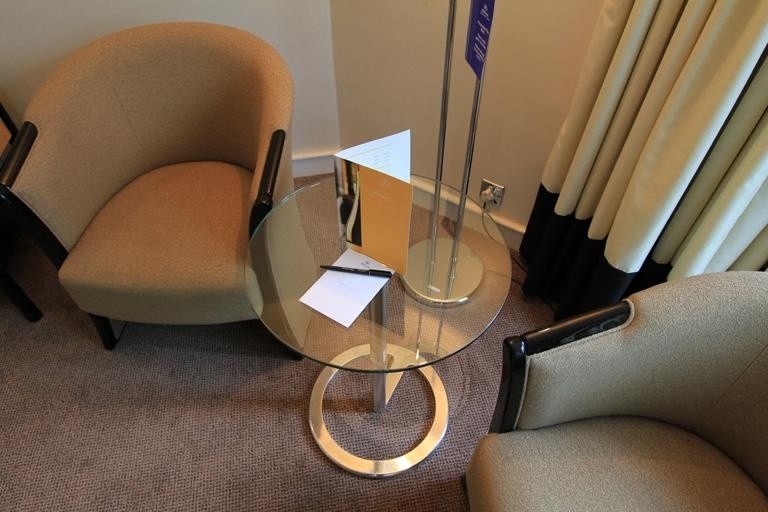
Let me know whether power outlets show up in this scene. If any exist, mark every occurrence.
[481,178,504,207]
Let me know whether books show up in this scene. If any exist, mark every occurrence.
[332,128,413,277]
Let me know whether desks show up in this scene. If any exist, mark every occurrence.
[245,173,513,478]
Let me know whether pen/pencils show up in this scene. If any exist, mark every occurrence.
[320,265,392,278]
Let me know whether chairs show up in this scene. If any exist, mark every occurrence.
[0,103,45,322]
[0,23,315,358]
[462,272,768,511]
